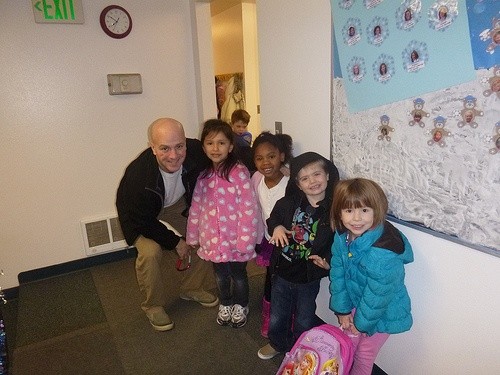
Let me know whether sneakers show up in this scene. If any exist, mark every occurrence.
[231,304,249,327]
[217,305,232,326]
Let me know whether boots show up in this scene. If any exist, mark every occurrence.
[260,296,271,338]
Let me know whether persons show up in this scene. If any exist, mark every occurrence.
[250,132,292,338]
[186,119,265,328]
[258,152,339,359]
[116,118,219,331]
[330,178,414,375]
[231,109,252,144]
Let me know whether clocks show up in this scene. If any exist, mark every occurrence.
[99,5,133,39]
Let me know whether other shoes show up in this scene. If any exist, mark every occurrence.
[181,293,219,307]
[258,344,279,360]
[141,305,174,331]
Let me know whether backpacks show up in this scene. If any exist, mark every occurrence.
[275,323,358,375]
